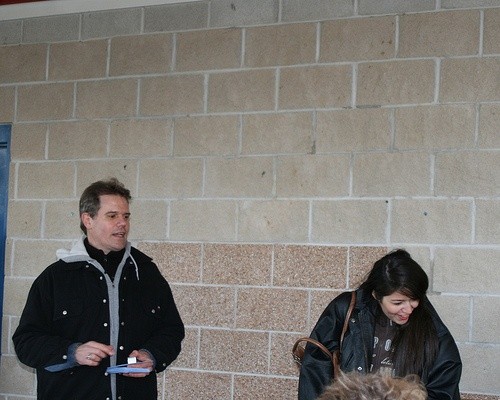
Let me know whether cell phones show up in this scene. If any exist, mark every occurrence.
[106,364,152,373]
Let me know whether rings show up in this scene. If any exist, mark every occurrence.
[87,353,93,360]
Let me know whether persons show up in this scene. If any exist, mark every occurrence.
[11,178,185,400]
[296,249,463,400]
